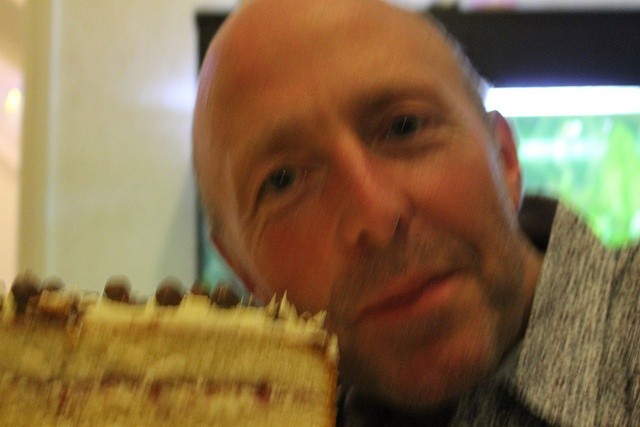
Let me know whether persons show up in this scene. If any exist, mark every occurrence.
[191,1,638,427]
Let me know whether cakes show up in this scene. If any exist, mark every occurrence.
[0,290,341,427]
